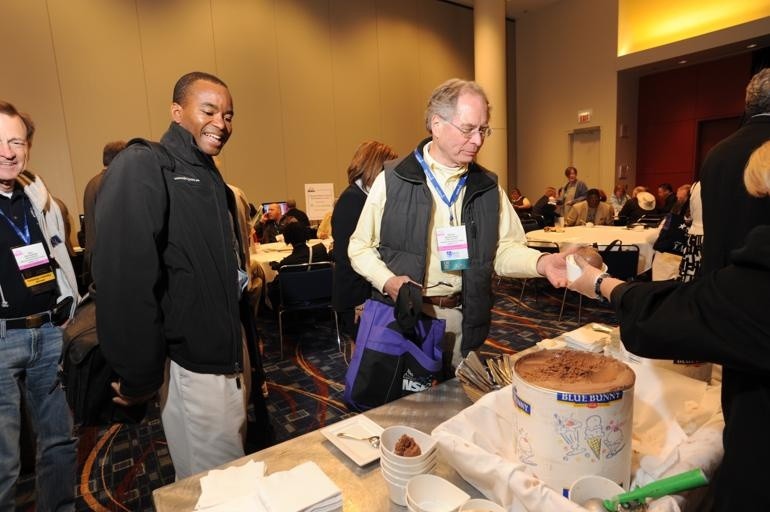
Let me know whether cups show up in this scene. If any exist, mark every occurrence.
[254,242,260,249]
[554,216,564,233]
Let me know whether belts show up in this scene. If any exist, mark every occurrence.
[7,313,50,331]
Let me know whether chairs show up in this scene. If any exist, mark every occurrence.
[269,261,342,358]
[515,208,640,324]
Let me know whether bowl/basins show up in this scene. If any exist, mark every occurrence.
[379,424,506,512]
[566,476,627,507]
[564,254,609,286]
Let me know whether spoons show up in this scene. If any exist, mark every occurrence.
[333,430,380,450]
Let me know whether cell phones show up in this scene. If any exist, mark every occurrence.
[265,213,269,217]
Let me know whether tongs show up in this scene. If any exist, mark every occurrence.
[456,351,511,393]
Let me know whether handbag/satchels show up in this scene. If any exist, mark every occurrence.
[58,298,149,428]
[344,283,446,412]
[654,214,688,256]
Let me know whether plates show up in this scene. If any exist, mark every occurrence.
[319,413,387,470]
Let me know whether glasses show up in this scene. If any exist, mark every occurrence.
[438,112,491,137]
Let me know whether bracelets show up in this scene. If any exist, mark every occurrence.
[594,275,608,302]
[355,307,365,311]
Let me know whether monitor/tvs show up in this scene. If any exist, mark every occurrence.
[262,201,288,215]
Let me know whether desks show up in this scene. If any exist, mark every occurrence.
[149,321,727,510]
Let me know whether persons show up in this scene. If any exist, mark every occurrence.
[88,71,249,480]
[682,141,712,278]
[84,142,129,269]
[701,64,770,275]
[315,198,340,242]
[344,77,587,414]
[266,220,329,308]
[330,138,399,334]
[253,198,286,245]
[508,166,692,232]
[0,97,79,512]
[282,198,311,228]
[212,155,253,268]
[567,143,769,510]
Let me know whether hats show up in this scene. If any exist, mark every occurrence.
[636,191,656,210]
